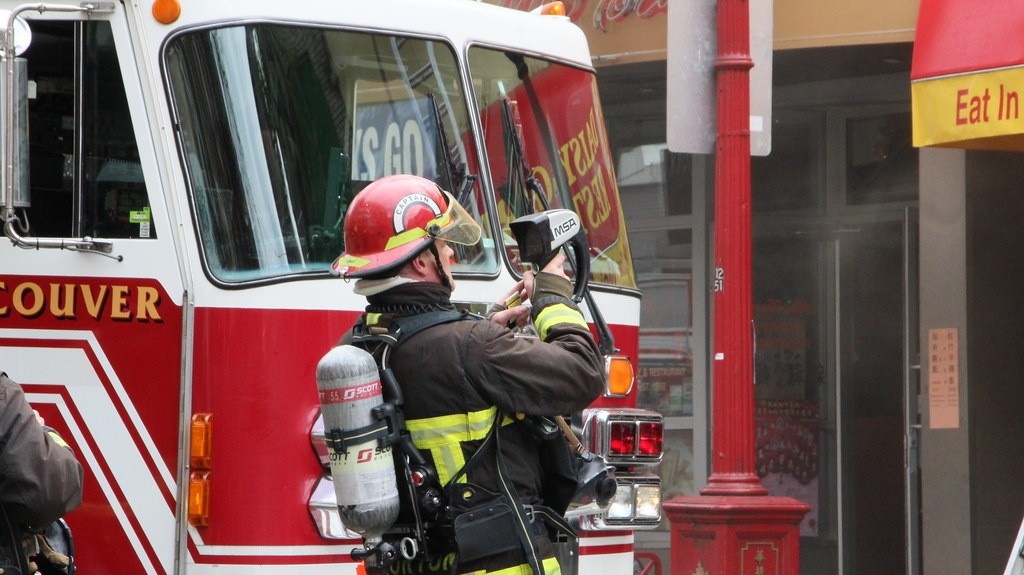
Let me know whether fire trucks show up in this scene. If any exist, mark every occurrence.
[0,3,666,575]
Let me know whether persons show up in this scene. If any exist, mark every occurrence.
[336,174,607,575]
[0,373,84,575]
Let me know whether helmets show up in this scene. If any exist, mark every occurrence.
[329,174,463,278]
